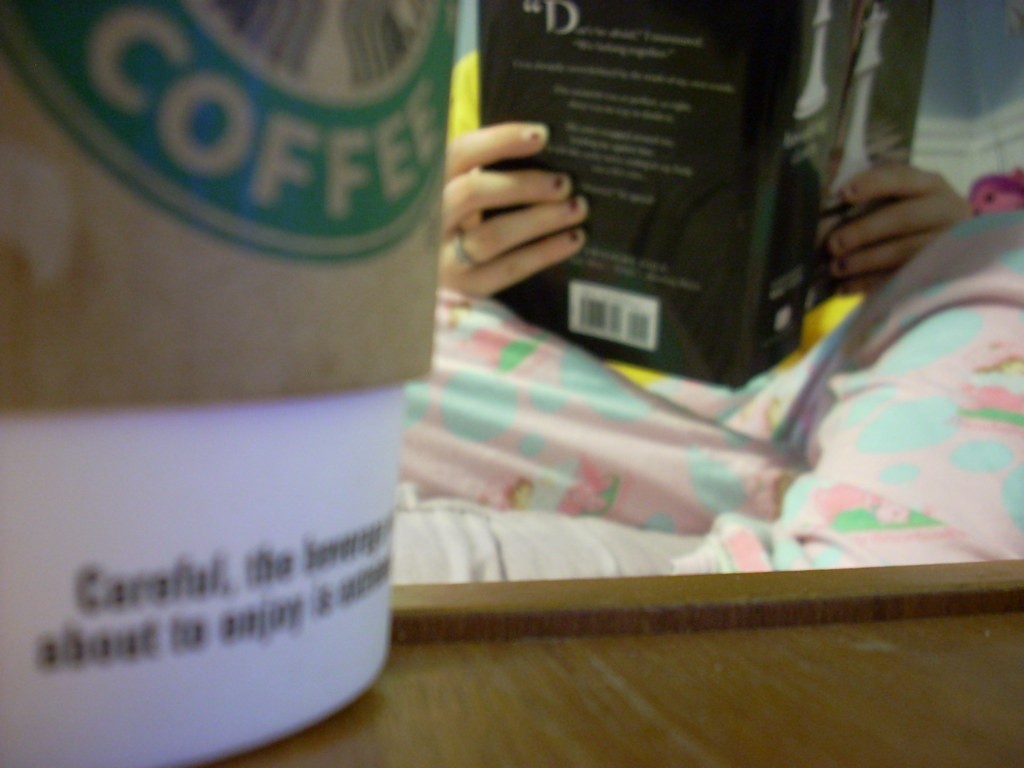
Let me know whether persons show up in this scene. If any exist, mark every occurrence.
[438,48,973,389]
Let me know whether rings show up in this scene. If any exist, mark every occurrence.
[454,232,475,269]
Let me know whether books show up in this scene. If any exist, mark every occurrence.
[477,0,937,393]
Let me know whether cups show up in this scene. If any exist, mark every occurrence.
[0,0,461,768]
